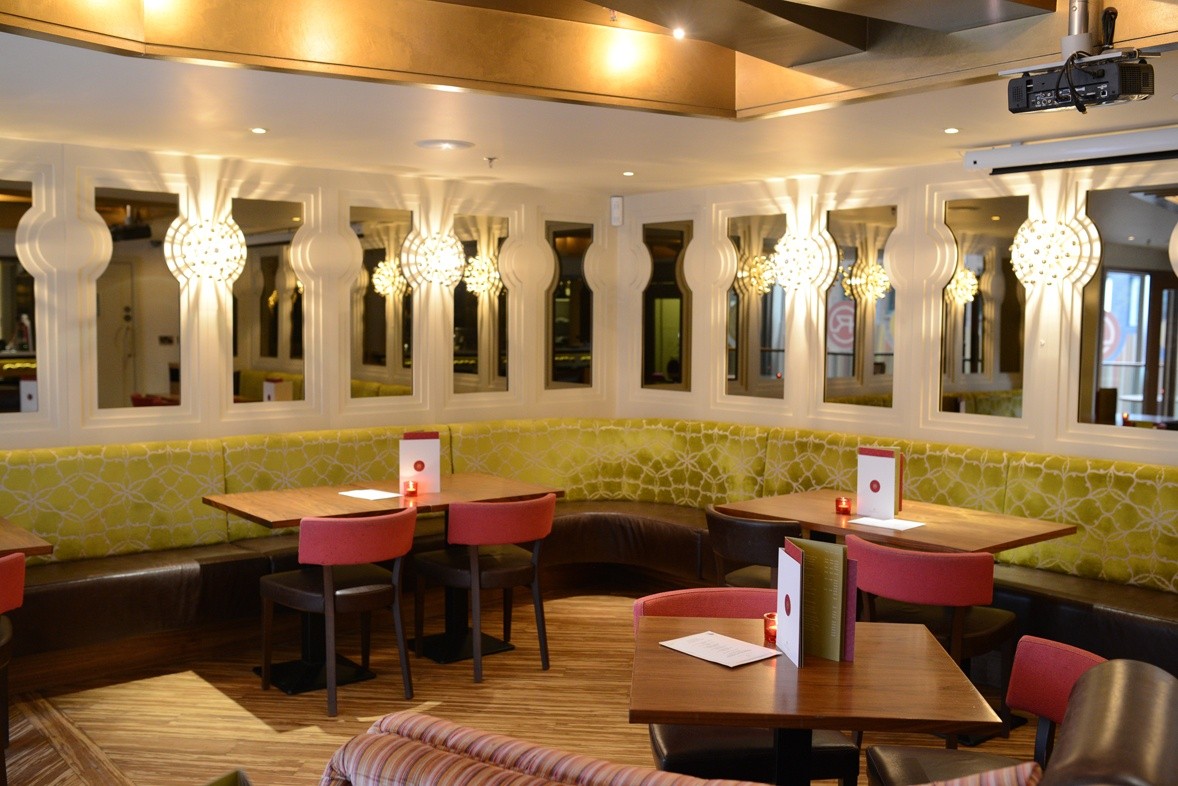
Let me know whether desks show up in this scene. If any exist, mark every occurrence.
[806,500,1090,748]
[625,605,1003,786]
[346,466,568,668]
[197,481,420,700]
[721,483,930,625]
[0,510,54,557]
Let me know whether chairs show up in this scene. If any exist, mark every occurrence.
[862,631,1105,786]
[630,582,862,786]
[409,493,556,680]
[252,499,420,721]
[1036,659,1178,786]
[701,499,811,586]
[839,528,1017,753]
[1,549,30,784]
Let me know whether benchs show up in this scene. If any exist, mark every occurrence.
[1,416,1177,686]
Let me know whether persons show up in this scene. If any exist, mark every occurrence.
[666,359,681,384]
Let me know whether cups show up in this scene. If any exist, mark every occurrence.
[764,612,778,642]
[404,480,418,497]
[837,497,852,514]
